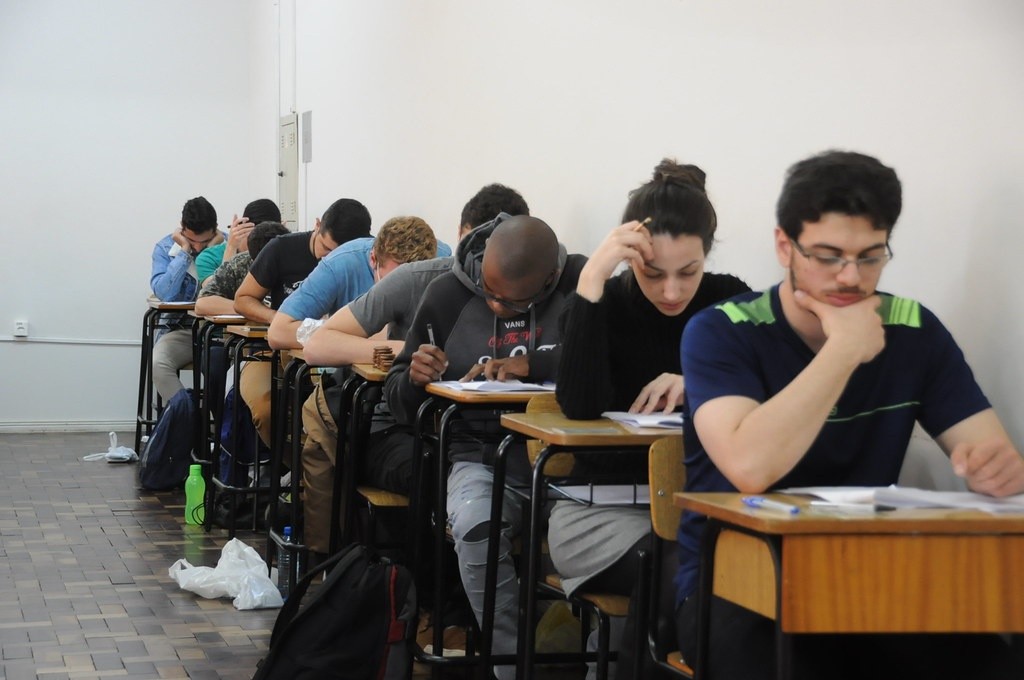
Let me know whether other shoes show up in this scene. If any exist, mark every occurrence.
[415,613,481,660]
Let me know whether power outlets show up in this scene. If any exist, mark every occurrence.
[13,320,27,337]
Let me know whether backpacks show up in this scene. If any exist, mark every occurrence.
[208,385,276,526]
[252,542,416,680]
[138,389,200,491]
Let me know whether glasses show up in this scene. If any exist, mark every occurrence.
[476,275,535,314]
[790,239,893,271]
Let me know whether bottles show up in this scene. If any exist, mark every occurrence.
[278,527,299,601]
[185,465,206,525]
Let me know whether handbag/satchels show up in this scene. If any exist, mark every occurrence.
[168,538,285,610]
[83,432,139,463]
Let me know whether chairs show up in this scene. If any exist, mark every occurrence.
[132,293,694,679]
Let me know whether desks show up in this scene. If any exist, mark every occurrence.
[187,309,204,464]
[204,315,250,526]
[135,302,196,457]
[482,409,682,680]
[206,326,268,541]
[264,347,327,595]
[329,363,390,556]
[670,491,1024,680]
[404,378,556,680]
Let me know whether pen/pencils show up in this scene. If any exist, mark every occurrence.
[428,322,443,382]
[737,495,801,514]
[228,221,246,228]
[632,217,653,232]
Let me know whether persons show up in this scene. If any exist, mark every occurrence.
[382,212,591,679]
[546,155,766,680]
[676,148,1024,680]
[150,184,532,635]
